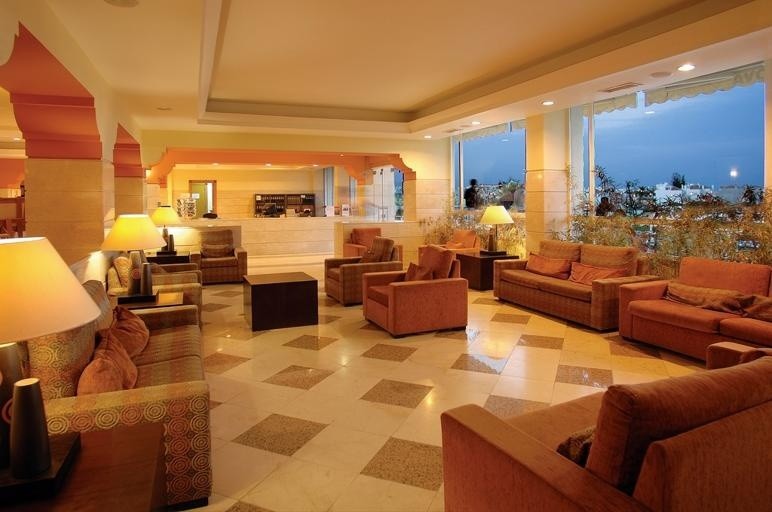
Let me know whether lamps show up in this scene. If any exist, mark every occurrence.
[99,214,168,303]
[478,206,514,256]
[151,205,182,255]
[0,236,102,491]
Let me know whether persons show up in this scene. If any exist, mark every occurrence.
[595,197,618,220]
[514,184,525,212]
[464,180,483,206]
[497,181,506,190]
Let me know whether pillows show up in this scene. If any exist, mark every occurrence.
[77,305,150,397]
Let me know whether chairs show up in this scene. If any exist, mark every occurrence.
[324,228,481,339]
[190,229,247,285]
[107,250,204,321]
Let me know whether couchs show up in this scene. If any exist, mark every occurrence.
[16,282,214,512]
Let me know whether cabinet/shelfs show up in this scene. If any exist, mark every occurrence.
[255,193,316,218]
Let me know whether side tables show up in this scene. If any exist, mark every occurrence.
[0,421,170,512]
[146,251,191,263]
